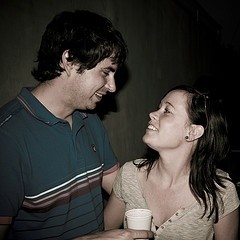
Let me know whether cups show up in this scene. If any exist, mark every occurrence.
[125,208,153,232]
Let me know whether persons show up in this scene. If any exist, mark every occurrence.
[103,83,240,240]
[0,10,154,240]
